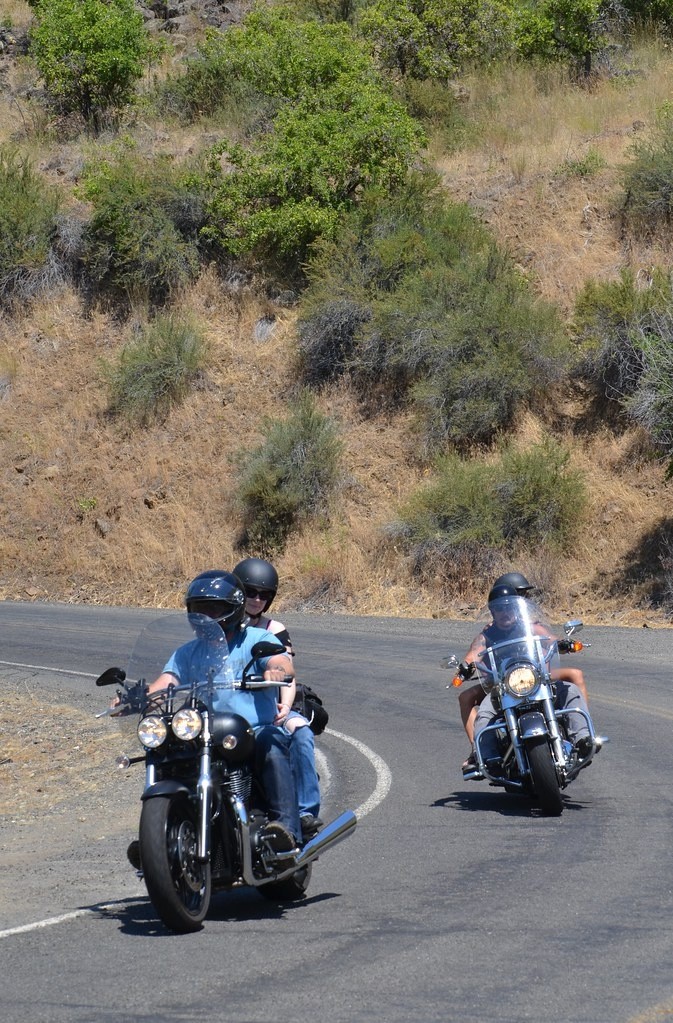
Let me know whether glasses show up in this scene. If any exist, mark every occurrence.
[493,605,514,611]
[245,587,273,600]
[518,591,527,596]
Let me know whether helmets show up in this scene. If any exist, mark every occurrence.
[493,572,535,593]
[488,585,517,607]
[185,570,246,640]
[232,559,278,613]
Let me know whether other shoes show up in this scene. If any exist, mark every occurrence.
[126,841,142,871]
[263,821,297,869]
[574,738,602,766]
[462,763,486,781]
[300,815,323,833]
[461,753,476,769]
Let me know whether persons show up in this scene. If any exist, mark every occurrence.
[458,572,602,778]
[233,558,323,833]
[111,570,302,871]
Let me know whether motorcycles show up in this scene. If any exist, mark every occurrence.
[95,615,358,934]
[438,596,609,820]
